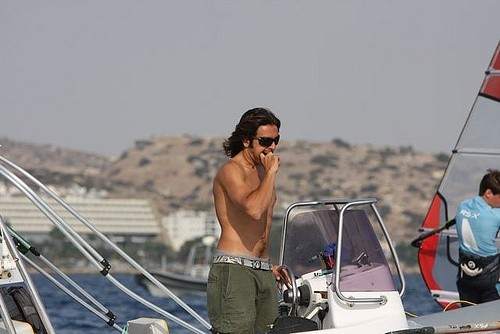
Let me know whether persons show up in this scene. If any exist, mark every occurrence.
[206,107,291,334]
[455,168,500,307]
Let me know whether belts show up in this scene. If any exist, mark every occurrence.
[212,256,271,272]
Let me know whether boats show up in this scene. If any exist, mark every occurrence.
[0,153,500,333]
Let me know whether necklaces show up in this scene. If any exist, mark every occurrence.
[243,151,257,169]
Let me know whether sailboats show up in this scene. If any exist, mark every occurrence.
[411,39,500,312]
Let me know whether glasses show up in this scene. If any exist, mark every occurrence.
[255,136,281,146]
